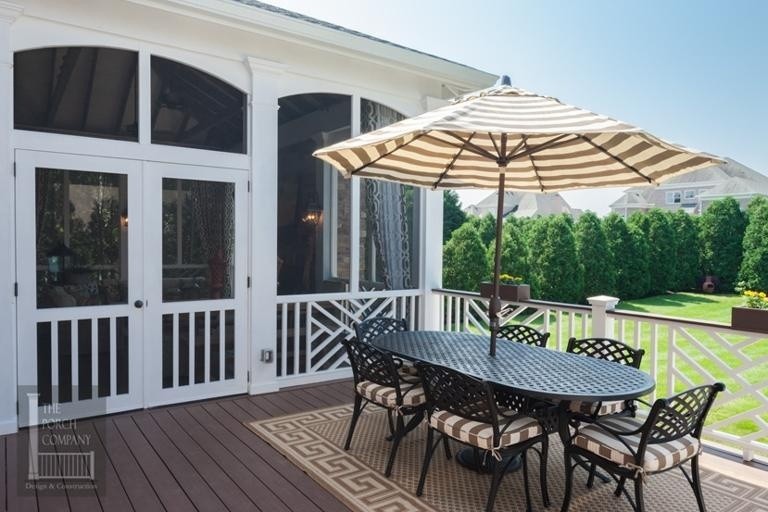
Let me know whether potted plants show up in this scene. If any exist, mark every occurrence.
[479,273,531,301]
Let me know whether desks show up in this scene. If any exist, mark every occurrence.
[375,330,655,482]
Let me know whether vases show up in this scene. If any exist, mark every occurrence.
[732,304,768,333]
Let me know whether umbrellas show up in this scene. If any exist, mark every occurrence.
[313,76,728,356]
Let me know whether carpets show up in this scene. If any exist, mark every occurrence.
[245,400,767,512]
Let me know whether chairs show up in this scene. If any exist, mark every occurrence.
[340,337,451,477]
[560,382,726,511]
[539,338,646,420]
[497,324,550,346]
[354,318,415,376]
[412,363,550,511]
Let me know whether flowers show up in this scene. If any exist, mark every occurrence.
[744,289,767,307]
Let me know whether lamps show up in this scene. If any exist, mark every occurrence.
[299,200,326,229]
